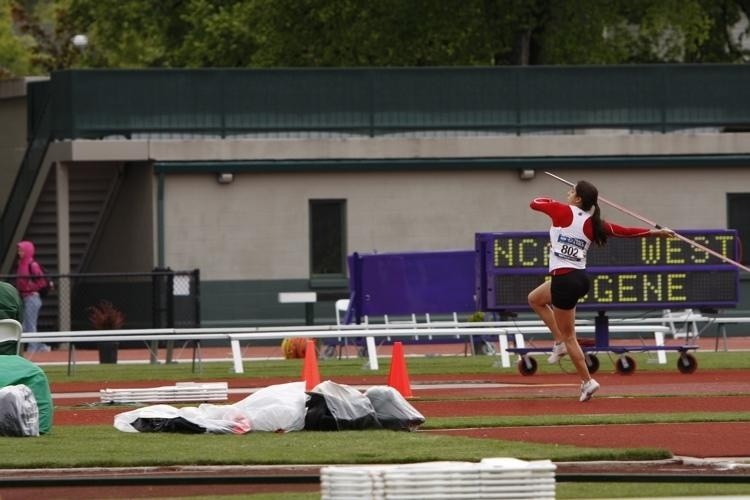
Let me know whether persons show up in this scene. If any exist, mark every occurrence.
[527,180,674,402]
[0,281,24,355]
[16,241,52,353]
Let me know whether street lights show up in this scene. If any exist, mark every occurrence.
[67,33,88,67]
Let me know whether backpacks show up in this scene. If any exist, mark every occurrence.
[27,257,54,297]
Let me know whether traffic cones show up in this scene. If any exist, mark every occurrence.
[299,341,323,391]
[388,342,417,404]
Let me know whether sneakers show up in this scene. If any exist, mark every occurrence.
[547,340,566,365]
[577,378,598,401]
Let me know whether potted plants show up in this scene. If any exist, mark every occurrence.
[89,301,126,364]
[469,312,487,355]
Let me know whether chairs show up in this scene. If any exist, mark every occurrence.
[335,298,366,360]
[0,319,23,356]
